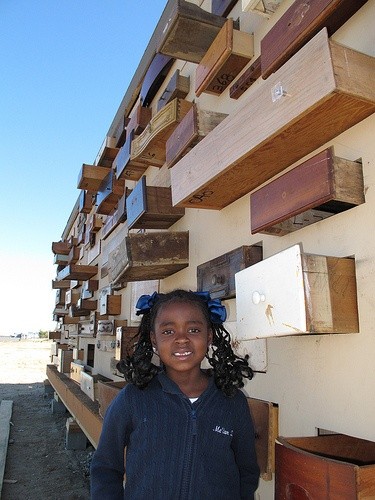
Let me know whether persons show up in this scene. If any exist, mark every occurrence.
[90,288,259,500]
